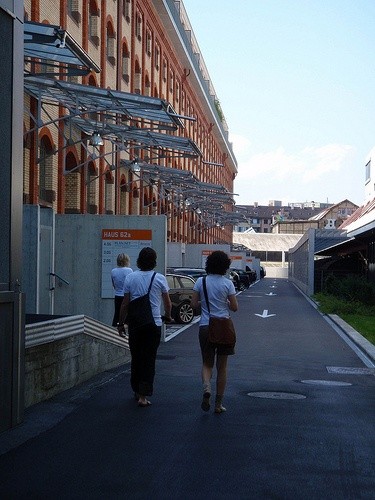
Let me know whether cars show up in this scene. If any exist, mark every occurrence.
[230,271,240,292]
[245,266,257,285]
[230,267,250,291]
[260,266,267,279]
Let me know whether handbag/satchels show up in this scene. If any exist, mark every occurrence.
[124,294,156,328]
[208,316,237,345]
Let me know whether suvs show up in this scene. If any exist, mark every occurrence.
[165,273,201,325]
[166,267,206,282]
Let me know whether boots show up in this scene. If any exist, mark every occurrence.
[215,394,228,412]
[201,385,211,412]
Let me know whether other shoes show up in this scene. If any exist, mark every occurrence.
[138,396,152,406]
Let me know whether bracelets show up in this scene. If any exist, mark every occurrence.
[117,322,124,327]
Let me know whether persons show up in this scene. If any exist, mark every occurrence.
[112,253,134,337]
[118,247,175,407]
[192,250,236,414]
